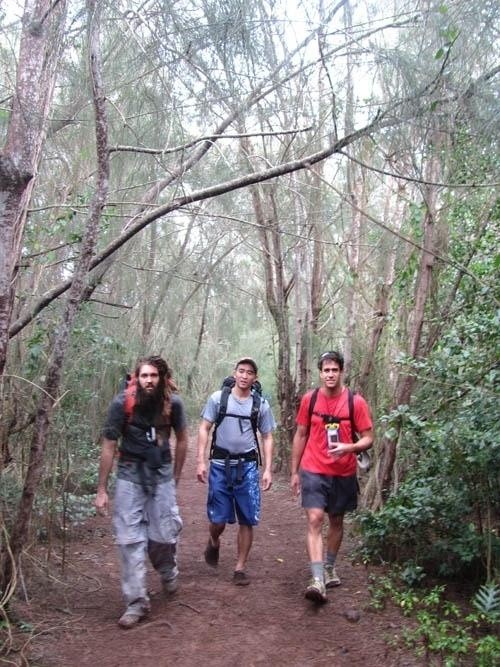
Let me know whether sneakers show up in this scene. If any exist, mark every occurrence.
[323,564,340,587]
[304,577,327,603]
[234,570,249,585]
[164,581,177,592]
[119,615,140,626]
[205,535,220,565]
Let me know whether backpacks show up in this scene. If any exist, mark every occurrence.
[123,371,171,439]
[215,375,262,432]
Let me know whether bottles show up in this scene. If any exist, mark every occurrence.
[325,422,339,449]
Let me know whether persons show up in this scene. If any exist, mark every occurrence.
[286,350,381,604]
[93,353,201,630]
[191,357,275,588]
